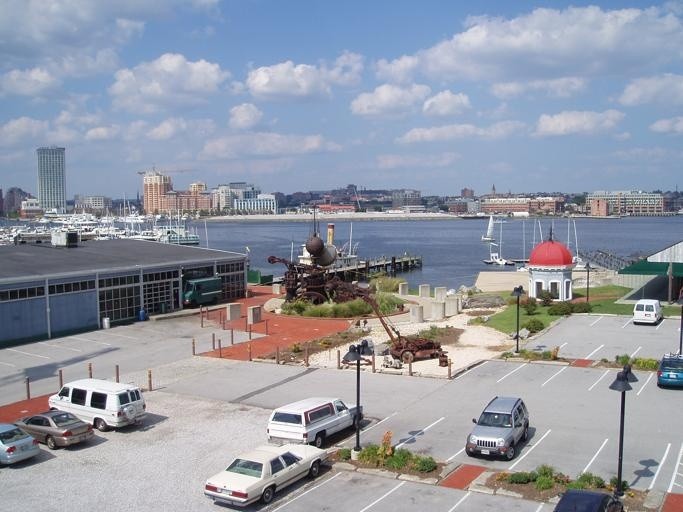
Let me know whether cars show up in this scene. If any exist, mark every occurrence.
[204,442,332,509]
[553,488,623,512]
[0,423,40,466]
[656,352,683,391]
[14,409,95,451]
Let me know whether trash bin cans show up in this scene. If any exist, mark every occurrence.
[102,318,110,329]
[139,311,144,321]
[161,303,165,313]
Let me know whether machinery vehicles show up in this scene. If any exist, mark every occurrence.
[328,279,443,363]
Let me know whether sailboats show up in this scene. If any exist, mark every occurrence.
[482,214,495,241]
[0,194,199,246]
[494,218,514,266]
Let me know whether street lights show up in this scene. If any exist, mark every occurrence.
[512,285,523,352]
[342,338,376,461]
[584,262,593,301]
[610,362,641,497]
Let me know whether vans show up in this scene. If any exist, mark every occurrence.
[632,297,663,326]
[49,378,147,433]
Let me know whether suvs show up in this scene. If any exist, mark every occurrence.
[466,394,530,461]
[266,395,365,448]
